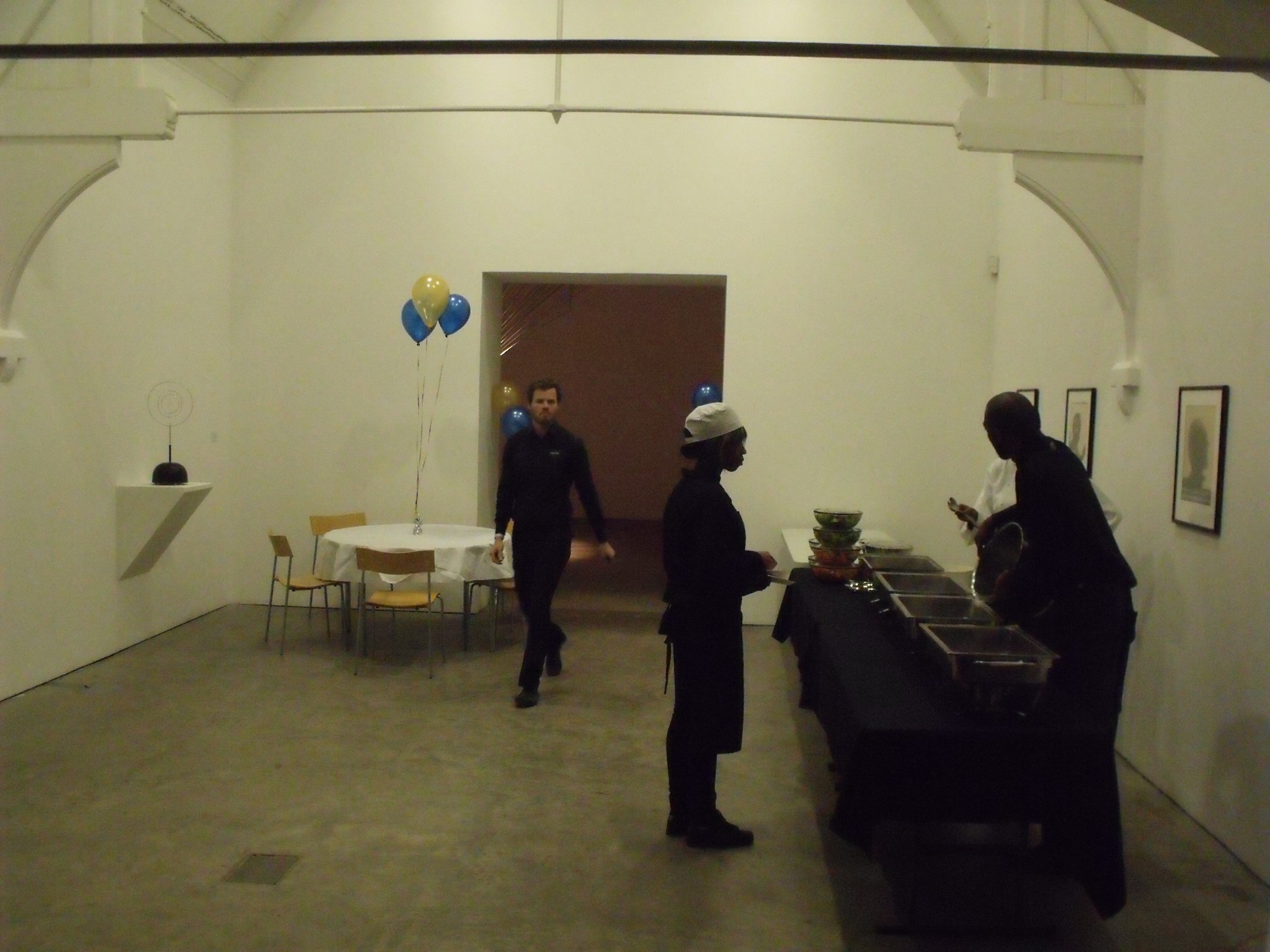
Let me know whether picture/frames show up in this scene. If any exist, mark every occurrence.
[1016,387,1040,408]
[1170,385,1229,537]
[1062,387,1098,479]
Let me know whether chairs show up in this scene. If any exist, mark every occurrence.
[457,519,527,651]
[350,545,446,675]
[307,510,396,638]
[266,525,346,654]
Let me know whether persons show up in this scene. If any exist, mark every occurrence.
[984,391,1137,918]
[1069,413,1086,457]
[489,380,616,707]
[948,454,1125,597]
[1183,418,1210,488]
[658,403,777,846]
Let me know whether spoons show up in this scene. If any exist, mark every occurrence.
[947,497,979,530]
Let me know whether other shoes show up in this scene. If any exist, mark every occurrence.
[545,639,563,676]
[515,690,542,709]
[665,808,739,838]
[685,815,755,848]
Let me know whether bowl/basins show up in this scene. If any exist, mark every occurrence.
[971,522,1030,596]
[807,509,862,584]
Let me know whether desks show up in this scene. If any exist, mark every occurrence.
[312,523,513,652]
[776,522,910,567]
[769,567,1109,948]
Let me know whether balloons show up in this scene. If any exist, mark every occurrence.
[492,383,520,412]
[690,382,721,409]
[412,275,448,326]
[402,299,437,347]
[501,406,532,436]
[438,296,471,336]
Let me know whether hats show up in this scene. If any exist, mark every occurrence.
[683,402,744,445]
